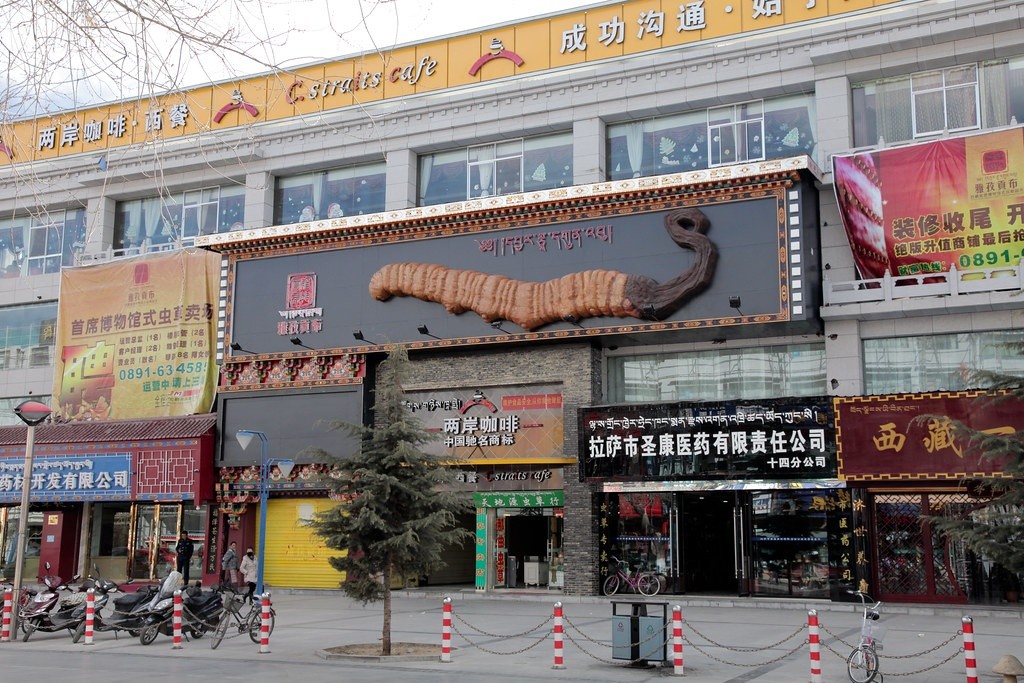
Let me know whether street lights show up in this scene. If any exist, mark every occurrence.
[236,429,295,609]
[3,401,52,647]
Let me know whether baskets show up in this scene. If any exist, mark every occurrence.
[608,564,618,575]
[223,594,245,612]
[860,617,887,643]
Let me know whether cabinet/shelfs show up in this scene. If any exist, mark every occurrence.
[524,562,548,586]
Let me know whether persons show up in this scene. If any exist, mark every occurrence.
[239,548,258,606]
[219,541,237,594]
[176,530,194,586]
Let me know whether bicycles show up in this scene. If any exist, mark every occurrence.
[9,582,39,633]
[602,555,660,596]
[209,582,277,651]
[845,587,883,683]
[626,561,669,593]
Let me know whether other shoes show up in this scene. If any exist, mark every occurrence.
[249,602,253,605]
[242,596,246,602]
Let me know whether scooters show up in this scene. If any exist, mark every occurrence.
[140,564,223,647]
[73,562,160,644]
[0,576,28,616]
[18,562,91,643]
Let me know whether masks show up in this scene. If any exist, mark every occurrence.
[247,552,253,557]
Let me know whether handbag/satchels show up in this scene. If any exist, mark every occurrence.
[222,557,225,571]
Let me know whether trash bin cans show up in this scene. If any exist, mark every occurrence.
[610,600,669,670]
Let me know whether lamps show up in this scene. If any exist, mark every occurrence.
[563,315,584,328]
[353,330,377,344]
[730,296,744,316]
[491,321,511,334]
[417,324,442,339]
[642,304,659,322]
[290,337,314,350]
[230,342,258,354]
